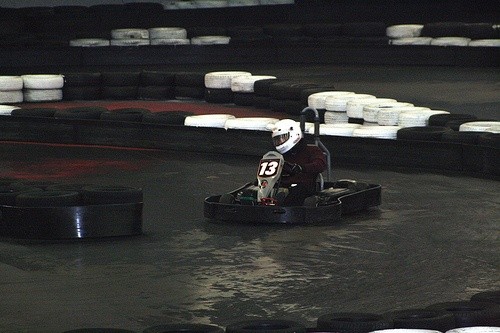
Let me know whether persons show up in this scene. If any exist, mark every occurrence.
[252,118,328,206]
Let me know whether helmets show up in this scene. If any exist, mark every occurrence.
[271,118,304,155]
